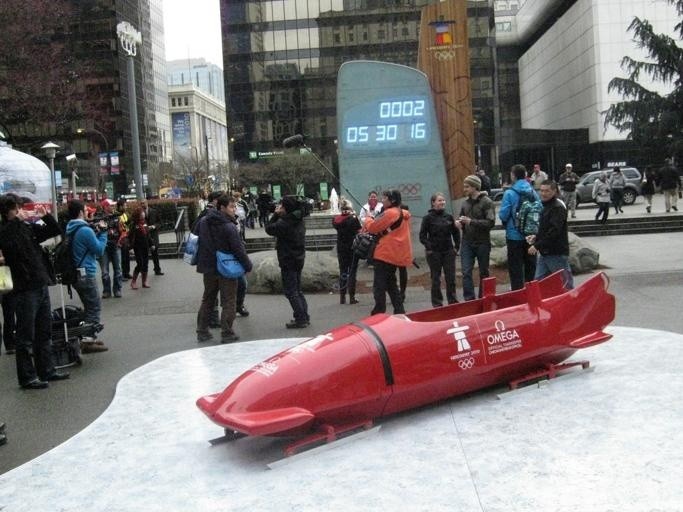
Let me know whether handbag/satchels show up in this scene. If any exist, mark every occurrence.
[216,250,245,279]
[352,233,376,260]
[0,266,14,292]
[183,233,200,265]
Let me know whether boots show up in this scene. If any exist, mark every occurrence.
[142,272,150,288]
[131,272,138,289]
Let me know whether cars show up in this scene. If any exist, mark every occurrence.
[490,189,504,201]
[283,194,324,211]
[101,186,189,207]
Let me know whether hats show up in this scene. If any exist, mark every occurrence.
[464,175,481,190]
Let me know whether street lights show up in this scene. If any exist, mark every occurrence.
[114,20,145,202]
[187,145,199,170]
[64,152,80,196]
[39,137,70,341]
[74,126,111,181]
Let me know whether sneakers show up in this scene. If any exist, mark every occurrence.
[286,319,310,328]
[197,333,212,340]
[80,340,108,353]
[221,335,239,343]
[114,291,121,297]
[237,306,249,315]
[102,291,110,298]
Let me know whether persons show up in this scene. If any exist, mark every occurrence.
[498,164,545,290]
[525,179,575,291]
[529,164,548,190]
[66,199,109,354]
[0,193,70,391]
[656,159,681,213]
[377,201,410,302]
[641,164,659,214]
[475,166,491,197]
[455,174,495,302]
[0,252,17,354]
[0,423,7,445]
[590,171,611,224]
[558,163,580,218]
[330,195,361,306]
[21,197,164,298]
[358,189,382,270]
[418,191,461,309]
[181,183,314,345]
[608,165,627,215]
[365,189,412,316]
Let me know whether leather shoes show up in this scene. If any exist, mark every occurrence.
[40,370,70,382]
[22,379,49,389]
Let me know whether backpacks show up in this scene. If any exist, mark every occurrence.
[50,225,87,285]
[506,187,544,237]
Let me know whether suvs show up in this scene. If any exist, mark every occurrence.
[554,166,644,208]
